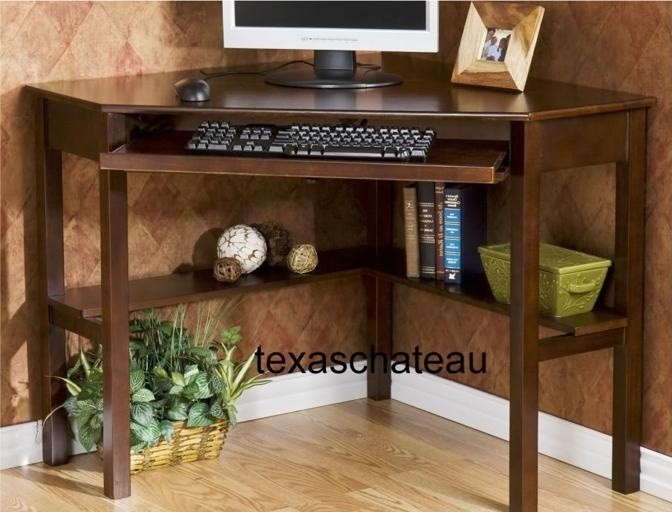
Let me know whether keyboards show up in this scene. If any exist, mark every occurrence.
[185,118,437,162]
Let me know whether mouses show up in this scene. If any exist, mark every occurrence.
[173,77,212,102]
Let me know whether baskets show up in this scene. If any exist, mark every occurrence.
[96,413,230,474]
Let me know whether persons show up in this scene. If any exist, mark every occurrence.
[483,29,505,60]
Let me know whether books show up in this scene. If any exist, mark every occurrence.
[401,185,421,278]
[444,190,480,284]
[418,182,436,279]
[433,183,445,279]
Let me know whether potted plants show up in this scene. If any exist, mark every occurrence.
[41,295,273,477]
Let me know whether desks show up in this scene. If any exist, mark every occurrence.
[23,50,659,507]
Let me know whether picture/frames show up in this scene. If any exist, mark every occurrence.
[449,2,545,93]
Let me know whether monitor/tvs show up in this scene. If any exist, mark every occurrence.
[222,0,439,90]
